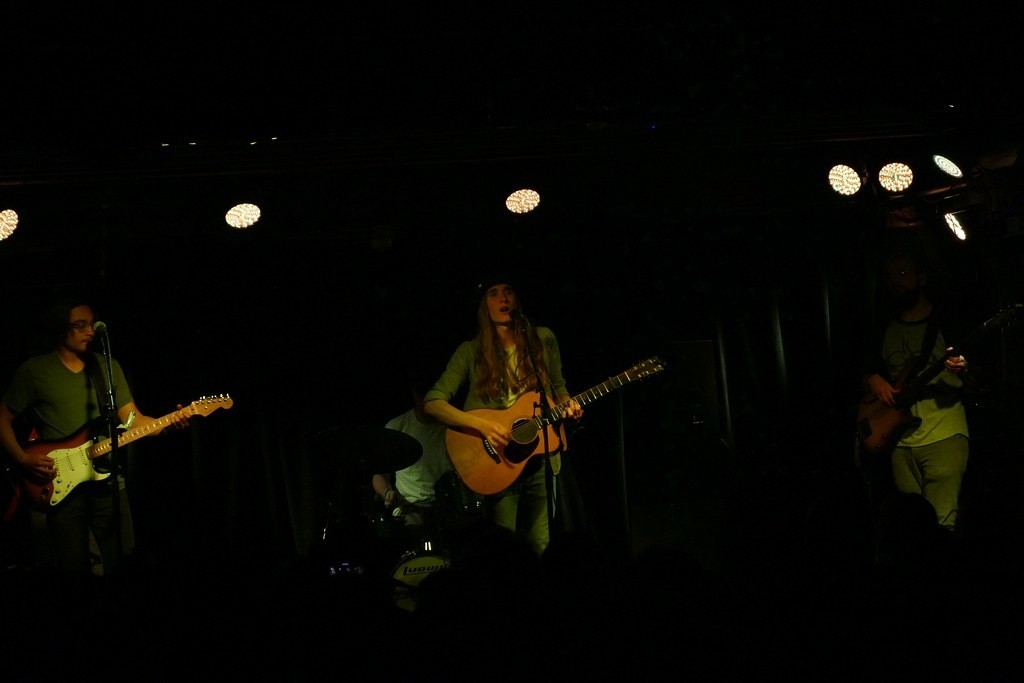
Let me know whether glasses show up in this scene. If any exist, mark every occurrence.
[883,269,920,279]
[68,319,96,332]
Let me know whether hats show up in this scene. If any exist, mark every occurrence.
[470,265,521,311]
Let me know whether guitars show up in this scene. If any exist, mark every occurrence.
[857,301,1023,450]
[18,392,235,507]
[445,352,669,496]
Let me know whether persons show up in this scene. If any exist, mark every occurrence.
[372,370,484,524]
[423,275,584,557]
[1,301,188,578]
[861,252,970,534]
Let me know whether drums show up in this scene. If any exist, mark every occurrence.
[390,543,452,612]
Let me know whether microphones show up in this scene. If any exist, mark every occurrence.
[509,309,528,335]
[94,322,107,355]
